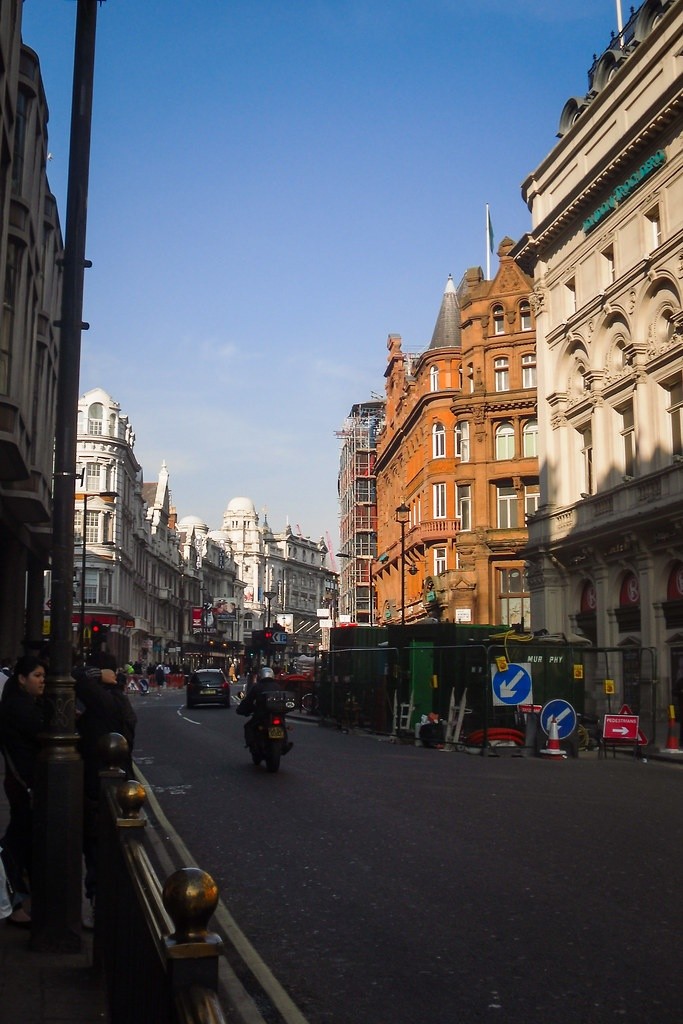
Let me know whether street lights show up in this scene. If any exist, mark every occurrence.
[210,640,214,666]
[334,552,374,628]
[223,643,228,668]
[78,490,121,664]
[394,501,412,628]
[262,591,278,667]
[237,604,241,661]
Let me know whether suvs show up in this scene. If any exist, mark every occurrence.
[183,668,234,708]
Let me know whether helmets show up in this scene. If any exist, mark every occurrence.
[258,667,274,682]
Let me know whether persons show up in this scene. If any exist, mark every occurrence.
[236,668,293,763]
[228,663,253,684]
[0,646,52,928]
[73,653,137,928]
[126,660,205,696]
[214,601,236,615]
[272,660,297,675]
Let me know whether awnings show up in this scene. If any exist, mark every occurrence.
[186,642,207,654]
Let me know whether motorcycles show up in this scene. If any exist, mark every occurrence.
[237,690,296,774]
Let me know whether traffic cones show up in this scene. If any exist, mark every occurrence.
[657,706,683,755]
[538,716,567,760]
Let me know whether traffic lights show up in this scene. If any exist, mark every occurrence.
[265,626,272,643]
[91,621,101,645]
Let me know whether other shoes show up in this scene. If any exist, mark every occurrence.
[6,913,32,931]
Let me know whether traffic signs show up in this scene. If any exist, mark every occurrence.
[602,713,641,741]
[270,631,288,645]
